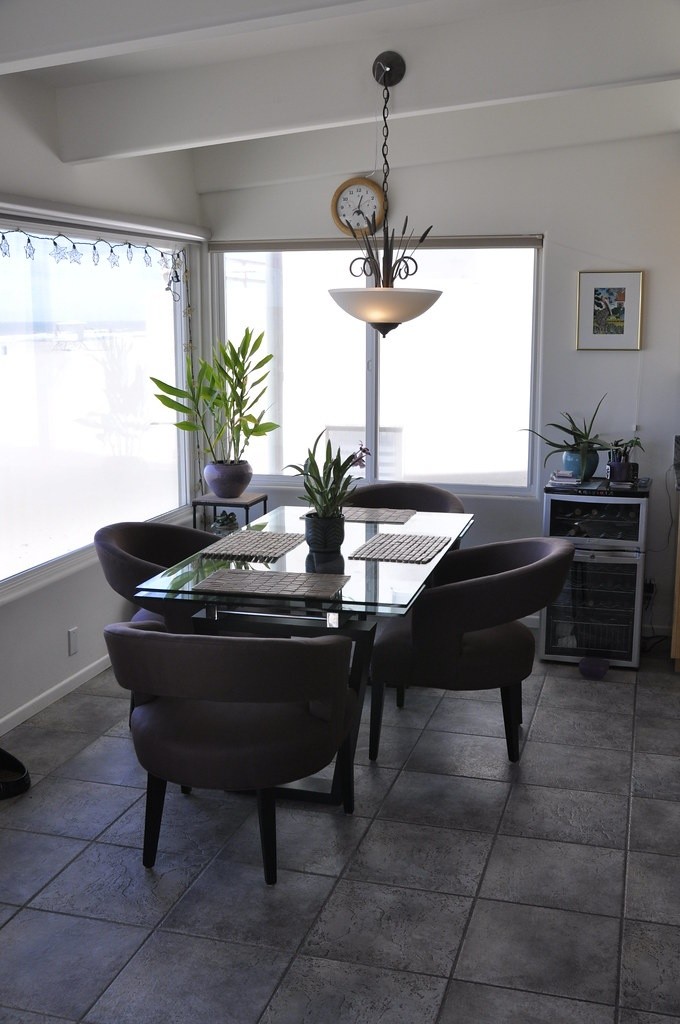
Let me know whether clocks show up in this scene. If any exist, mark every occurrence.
[331,178,388,238]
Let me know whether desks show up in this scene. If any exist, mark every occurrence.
[134,506,474,805]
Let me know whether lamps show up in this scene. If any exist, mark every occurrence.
[328,51,443,338]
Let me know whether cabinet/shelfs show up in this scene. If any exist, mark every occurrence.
[192,492,268,532]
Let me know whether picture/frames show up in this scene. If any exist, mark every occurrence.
[576,270,644,350]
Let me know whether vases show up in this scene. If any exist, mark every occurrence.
[306,511,345,553]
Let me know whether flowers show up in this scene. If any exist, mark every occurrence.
[281,428,372,518]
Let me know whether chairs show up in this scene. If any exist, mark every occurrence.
[340,484,465,551]
[103,621,357,885]
[369,536,576,762]
[94,522,291,637]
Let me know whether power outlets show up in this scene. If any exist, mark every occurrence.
[643,582,655,604]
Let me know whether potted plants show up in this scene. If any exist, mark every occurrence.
[150,326,280,498]
[519,393,621,481]
